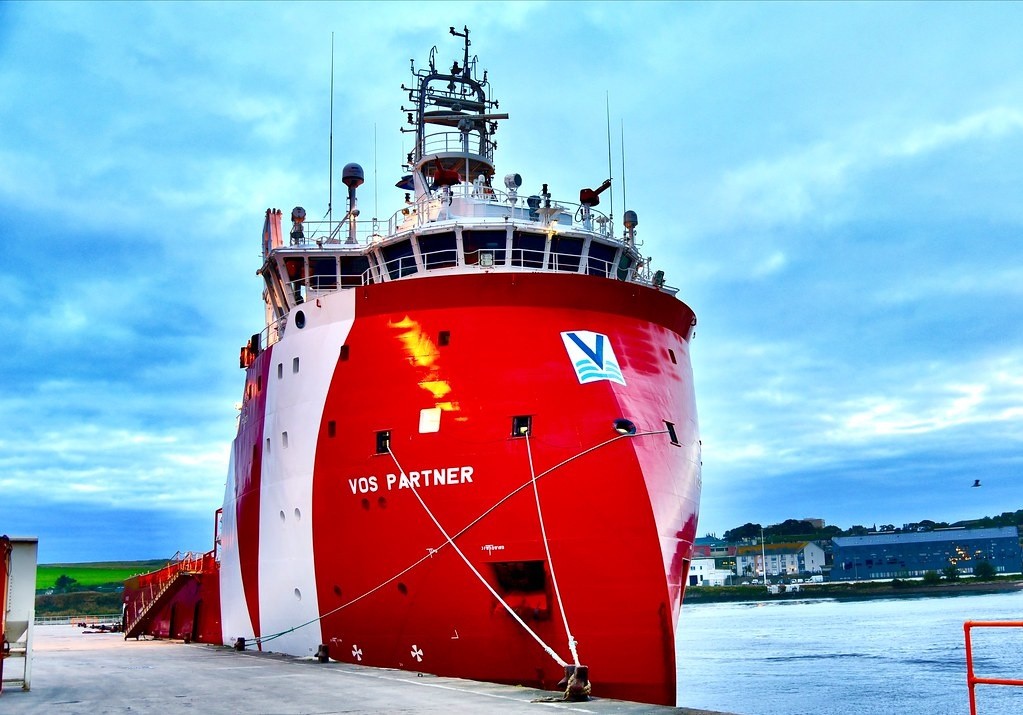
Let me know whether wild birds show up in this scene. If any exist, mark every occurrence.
[971,480,981,488]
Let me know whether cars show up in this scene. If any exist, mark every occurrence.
[742,575,823,585]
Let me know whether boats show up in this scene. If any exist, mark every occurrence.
[120,26,702,706]
